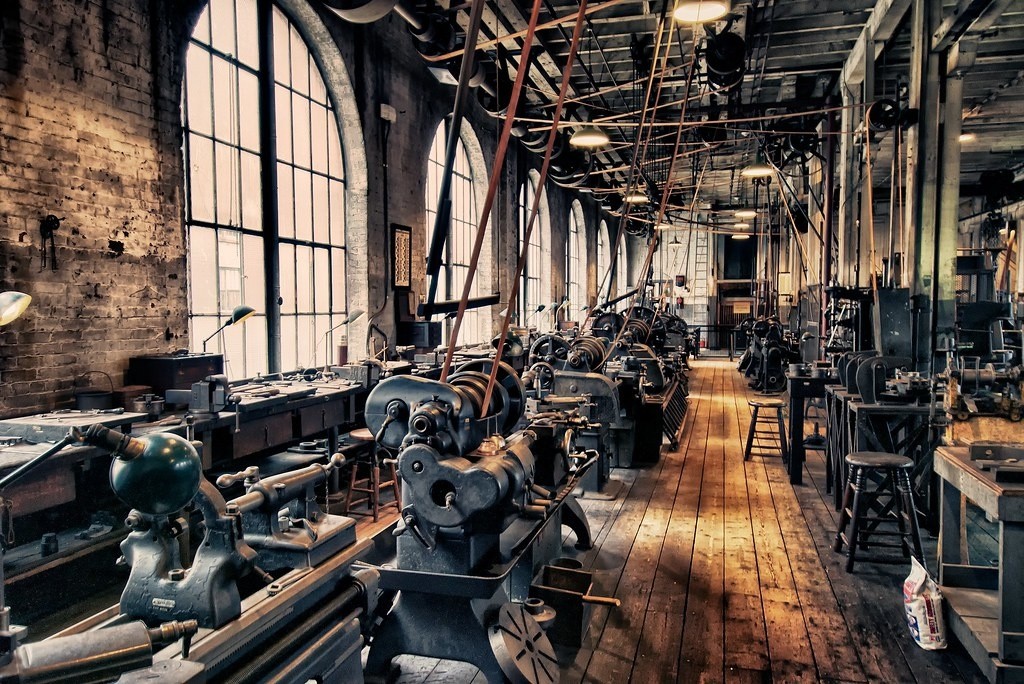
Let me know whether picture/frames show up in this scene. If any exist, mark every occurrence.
[390,222,411,291]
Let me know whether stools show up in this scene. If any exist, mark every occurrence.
[744,397,788,464]
[343,428,401,522]
[833,451,927,574]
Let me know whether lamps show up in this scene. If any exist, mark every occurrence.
[539,302,558,332]
[734,178,756,217]
[525,305,545,326]
[555,300,571,332]
[0,291,33,326]
[674,0,731,22]
[622,183,648,202]
[569,30,609,146]
[322,309,365,376]
[572,306,589,321]
[740,147,774,177]
[437,312,457,324]
[204,305,256,352]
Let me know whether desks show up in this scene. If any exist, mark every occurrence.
[933,446,1024,684]
[824,383,946,540]
[784,371,841,486]
[0,343,548,606]
